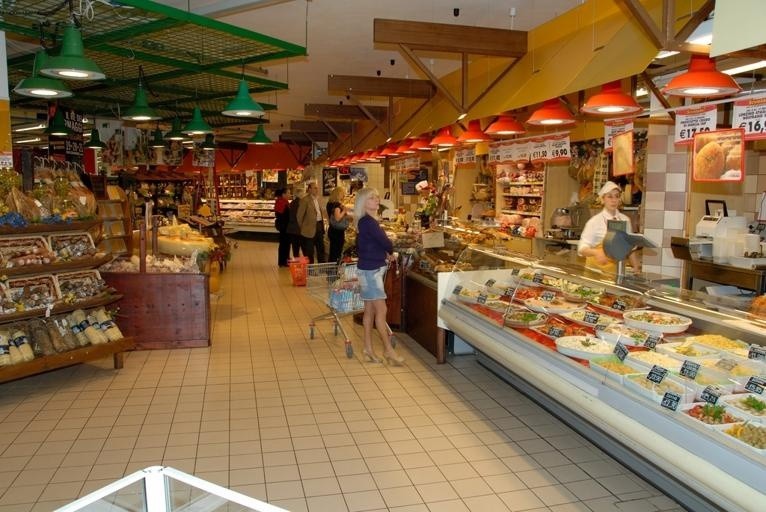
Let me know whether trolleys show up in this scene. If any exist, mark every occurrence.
[300,252,403,361]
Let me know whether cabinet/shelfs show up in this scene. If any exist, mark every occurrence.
[118,171,192,222]
[438,225,766,512]
[0,219,135,383]
[495,161,581,223]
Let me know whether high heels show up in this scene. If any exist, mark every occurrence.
[361,348,405,367]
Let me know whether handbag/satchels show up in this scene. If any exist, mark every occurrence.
[329,214,349,230]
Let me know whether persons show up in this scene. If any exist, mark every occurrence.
[296,182,326,276]
[354,188,405,366]
[290,189,308,257]
[577,181,632,265]
[326,187,348,284]
[616,176,642,206]
[140,197,150,217]
[274,187,292,268]
[415,180,441,228]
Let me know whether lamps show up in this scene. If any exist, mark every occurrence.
[121,65,162,121]
[45,99,75,136]
[580,80,643,115]
[322,119,494,168]
[84,117,107,149]
[221,63,266,117]
[525,97,576,126]
[247,117,273,145]
[39,0,106,82]
[483,110,528,135]
[152,87,216,150]
[14,24,76,99]
[664,54,742,98]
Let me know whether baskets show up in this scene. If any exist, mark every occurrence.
[288,256,310,285]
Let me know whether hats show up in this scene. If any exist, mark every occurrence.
[597,181,621,197]
[415,180,428,191]
